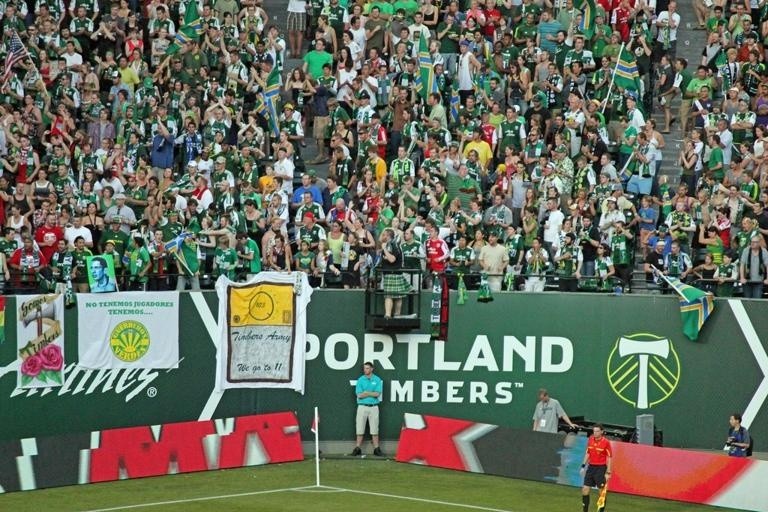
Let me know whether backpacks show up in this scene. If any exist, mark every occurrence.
[730,427,754,456]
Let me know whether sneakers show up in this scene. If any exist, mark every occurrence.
[351,447,361,456]
[374,447,382,456]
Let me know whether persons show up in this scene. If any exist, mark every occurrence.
[350,362,384,455]
[578,422,614,511]
[527,388,581,434]
[377,228,414,320]
[1,0,768,306]
[727,413,751,457]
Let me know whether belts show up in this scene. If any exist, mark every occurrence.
[361,404,378,407]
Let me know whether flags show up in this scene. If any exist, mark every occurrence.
[0,295,7,347]
[311,413,324,435]
[213,271,315,394]
[427,268,451,342]
[596,482,608,511]
[13,293,65,389]
[74,289,182,369]
[652,266,716,342]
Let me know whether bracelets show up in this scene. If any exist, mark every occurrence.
[580,462,586,468]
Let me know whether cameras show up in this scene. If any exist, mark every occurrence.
[726,438,738,446]
